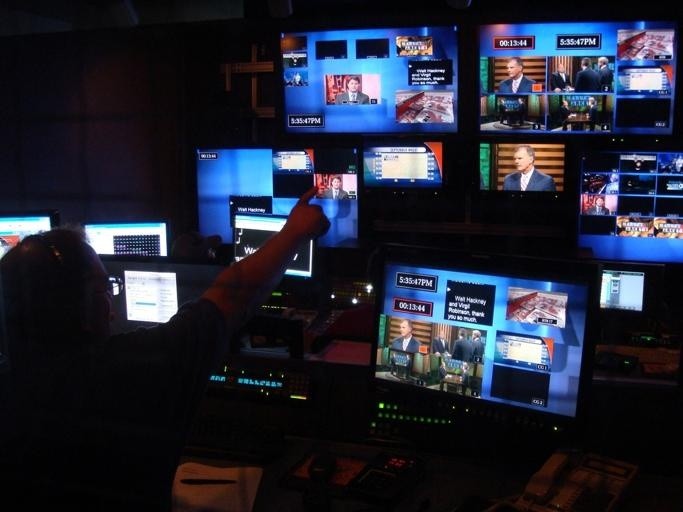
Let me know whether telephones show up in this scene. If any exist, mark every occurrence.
[515,449,640,512]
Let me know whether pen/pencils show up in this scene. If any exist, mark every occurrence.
[180,479,238,484]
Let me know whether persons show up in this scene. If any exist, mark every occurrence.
[334,77,369,104]
[389,318,485,394]
[503,147,554,192]
[0,183,334,512]
[585,194,612,215]
[498,56,613,131]
[319,176,353,198]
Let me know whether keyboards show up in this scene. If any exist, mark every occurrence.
[593,350,638,376]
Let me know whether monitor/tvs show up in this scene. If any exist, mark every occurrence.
[370,251,595,425]
[595,267,648,317]
[82,219,172,279]
[121,267,181,326]
[193,144,363,253]
[574,146,683,267]
[233,213,318,280]
[475,18,683,138]
[359,139,446,195]
[275,19,461,137]
[476,138,567,197]
[0,211,55,262]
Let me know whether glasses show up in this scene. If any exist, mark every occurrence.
[106,276,124,297]
